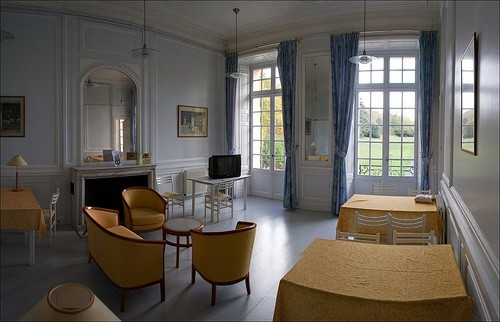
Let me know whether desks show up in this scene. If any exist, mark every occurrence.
[188,173,252,220]
[161,218,203,268]
[90,156,106,162]
[305,154,330,161]
[0,188,47,265]
[335,193,442,244]
[271,237,475,322]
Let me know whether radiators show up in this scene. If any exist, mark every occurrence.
[183,167,208,197]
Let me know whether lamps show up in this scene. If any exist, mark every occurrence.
[130,0,162,58]
[6,155,28,191]
[348,0,379,64]
[84,76,96,88]
[224,8,248,78]
[310,141,317,155]
[20,283,124,322]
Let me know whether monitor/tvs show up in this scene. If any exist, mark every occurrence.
[208,154,241,179]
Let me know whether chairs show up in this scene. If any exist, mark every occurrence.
[336,184,436,245]
[25,188,61,247]
[82,175,255,311]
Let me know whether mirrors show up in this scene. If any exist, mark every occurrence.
[302,53,332,166]
[79,65,142,165]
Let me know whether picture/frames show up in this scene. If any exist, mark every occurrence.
[460,32,479,155]
[111,150,121,166]
[0,95,25,137]
[177,105,208,137]
